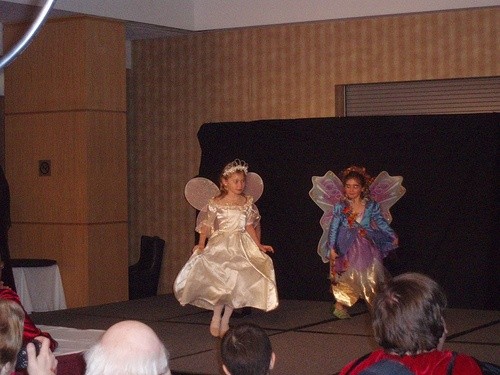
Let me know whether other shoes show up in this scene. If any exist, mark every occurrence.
[333,305,347,319]
[220,320,230,335]
[210,321,221,337]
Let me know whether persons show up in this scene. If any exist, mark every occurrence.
[310,166,407,319]
[0,261,172,375]
[220,322,276,375]
[173,158,279,339]
[338,274,500,375]
